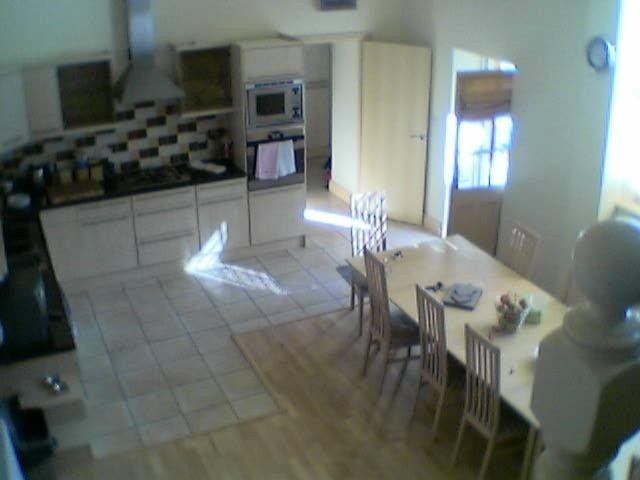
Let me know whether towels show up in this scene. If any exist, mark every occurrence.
[279,139,296,176]
[254,141,279,181]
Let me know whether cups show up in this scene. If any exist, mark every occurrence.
[493,288,532,337]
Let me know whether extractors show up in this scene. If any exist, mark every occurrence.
[112,63,187,107]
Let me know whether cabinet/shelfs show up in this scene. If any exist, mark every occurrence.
[24,55,119,141]
[233,38,310,253]
[177,42,237,119]
[39,176,141,285]
[189,178,251,255]
[130,183,201,269]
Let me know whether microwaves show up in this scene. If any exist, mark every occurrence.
[247,84,304,130]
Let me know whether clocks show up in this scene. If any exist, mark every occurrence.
[586,37,614,72]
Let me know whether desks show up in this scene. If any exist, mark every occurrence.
[342,232,575,479]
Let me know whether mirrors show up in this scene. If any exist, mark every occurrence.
[447,45,518,258]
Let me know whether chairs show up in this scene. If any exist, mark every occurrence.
[360,244,440,395]
[501,220,541,280]
[405,282,502,456]
[349,188,389,312]
[450,322,543,476]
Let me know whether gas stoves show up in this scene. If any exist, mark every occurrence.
[124,161,194,193]
